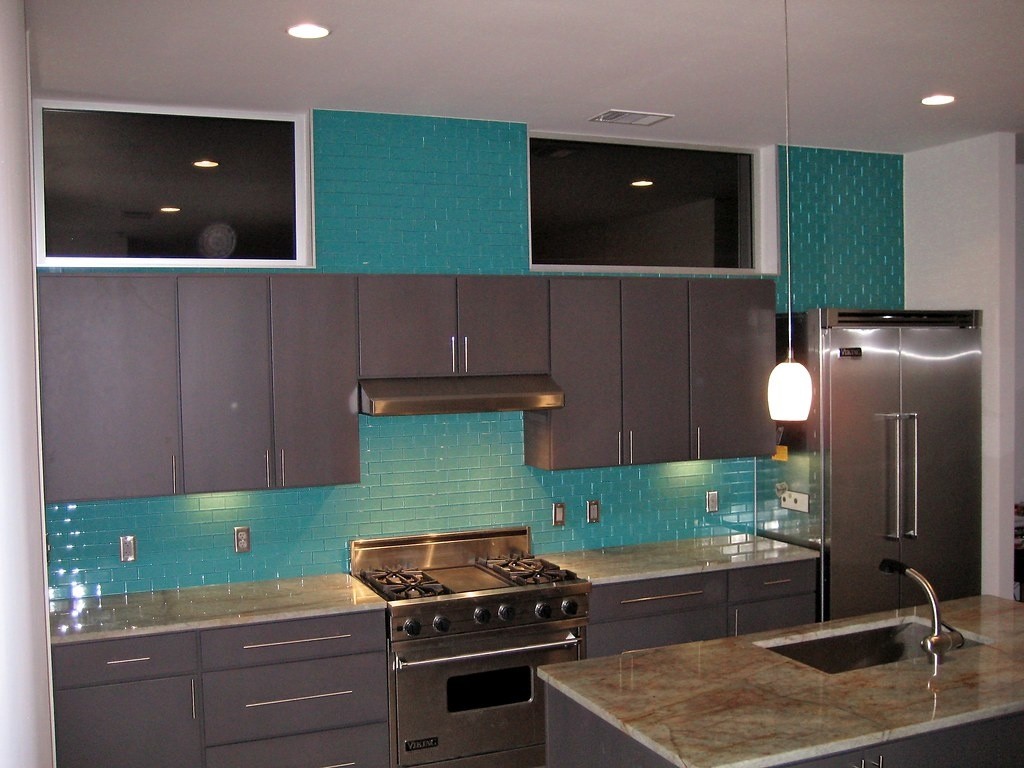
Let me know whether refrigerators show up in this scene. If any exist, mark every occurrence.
[776,304,981,623]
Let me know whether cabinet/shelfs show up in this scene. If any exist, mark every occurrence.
[538,532,820,660]
[49,574,390,767]
[540,593,1024,768]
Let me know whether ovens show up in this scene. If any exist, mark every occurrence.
[390,595,592,767]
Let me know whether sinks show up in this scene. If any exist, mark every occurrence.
[753,615,996,675]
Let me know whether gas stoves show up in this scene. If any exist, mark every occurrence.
[357,551,593,608]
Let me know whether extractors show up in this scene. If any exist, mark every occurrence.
[358,374,566,415]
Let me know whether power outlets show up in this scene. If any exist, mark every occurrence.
[552,502,565,526]
[705,490,719,513]
[585,499,600,524]
[233,526,251,552]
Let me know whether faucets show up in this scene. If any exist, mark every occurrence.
[878,557,965,665]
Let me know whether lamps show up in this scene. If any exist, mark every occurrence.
[767,351,814,422]
